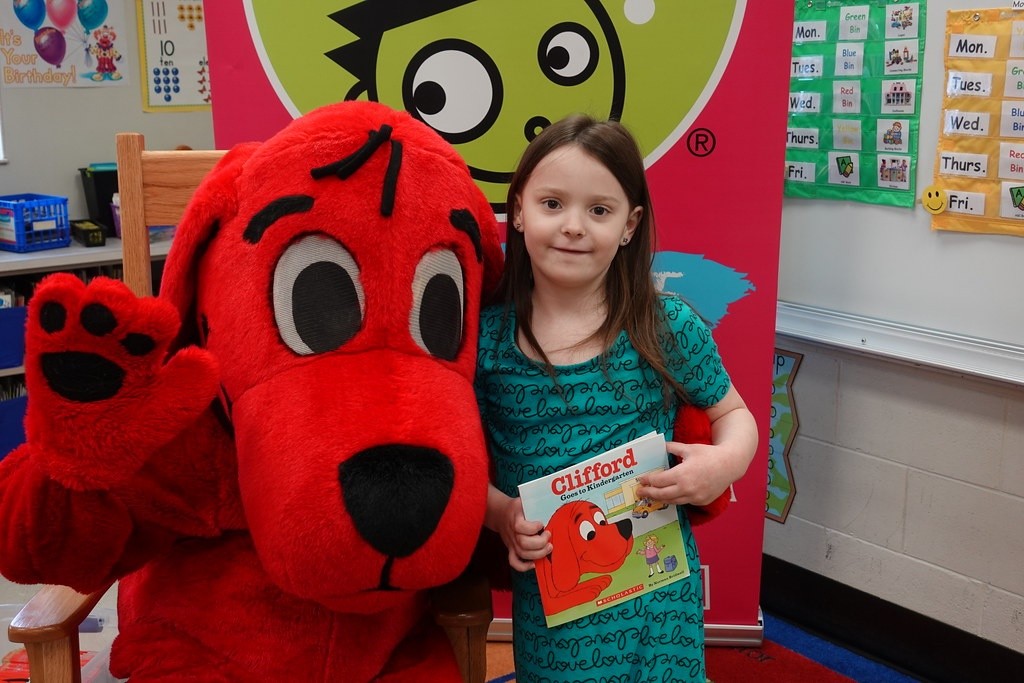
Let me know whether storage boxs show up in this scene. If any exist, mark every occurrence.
[0,193,72,253]
[0,305,27,369]
[0,396,27,462]
[69,219,107,248]
[78,162,119,238]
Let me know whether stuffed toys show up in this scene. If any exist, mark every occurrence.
[0,101,510,683]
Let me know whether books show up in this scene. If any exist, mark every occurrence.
[517,429,689,629]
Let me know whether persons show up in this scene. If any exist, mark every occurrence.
[473,112,761,683]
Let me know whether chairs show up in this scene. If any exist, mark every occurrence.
[0,131,495,683]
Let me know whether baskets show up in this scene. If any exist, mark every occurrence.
[70,219,106,247]
[0,192,71,253]
